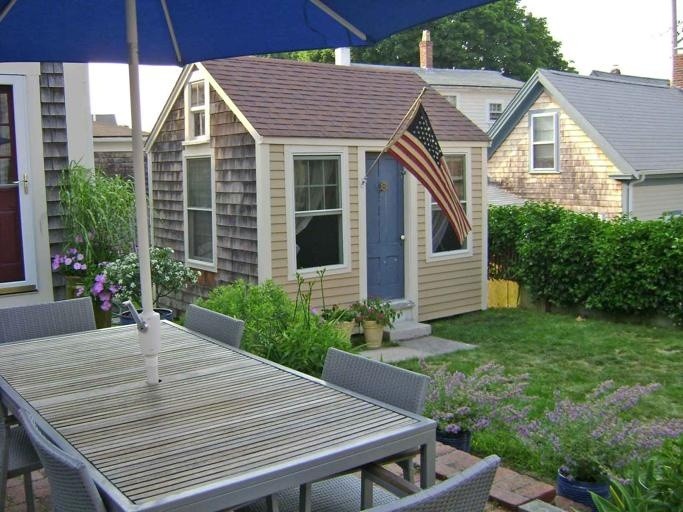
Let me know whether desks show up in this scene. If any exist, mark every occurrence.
[1,320,437,511]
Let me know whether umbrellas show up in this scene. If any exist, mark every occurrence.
[0,0,496,383]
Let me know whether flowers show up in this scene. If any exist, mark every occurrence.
[49,232,201,312]
[413,351,683,481]
[340,299,403,324]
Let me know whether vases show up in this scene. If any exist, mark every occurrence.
[118,308,172,325]
[556,465,612,512]
[361,320,384,349]
[64,276,111,330]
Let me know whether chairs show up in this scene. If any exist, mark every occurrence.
[298,346,430,510]
[0,298,95,342]
[364,455,499,511]
[185,303,244,349]
[16,408,105,510]
[1,401,44,511]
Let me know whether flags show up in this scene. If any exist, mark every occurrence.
[384,98,472,247]
